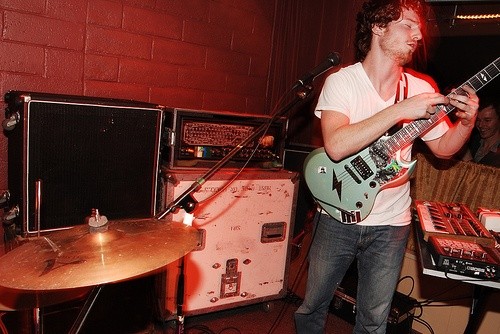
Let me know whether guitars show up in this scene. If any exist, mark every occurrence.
[303,57,500,225]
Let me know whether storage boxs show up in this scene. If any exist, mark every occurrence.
[157,168,299,318]
[329,281,416,334]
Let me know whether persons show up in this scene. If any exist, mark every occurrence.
[292,0,479,334]
[458,95,500,168]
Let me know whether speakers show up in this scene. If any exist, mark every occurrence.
[4,89,165,236]
[281,142,319,236]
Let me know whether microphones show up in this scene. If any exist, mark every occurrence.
[292,52,342,90]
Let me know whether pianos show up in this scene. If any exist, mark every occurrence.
[414,198,495,248]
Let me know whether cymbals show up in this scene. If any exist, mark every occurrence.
[0,218,200,290]
[0,286,91,311]
